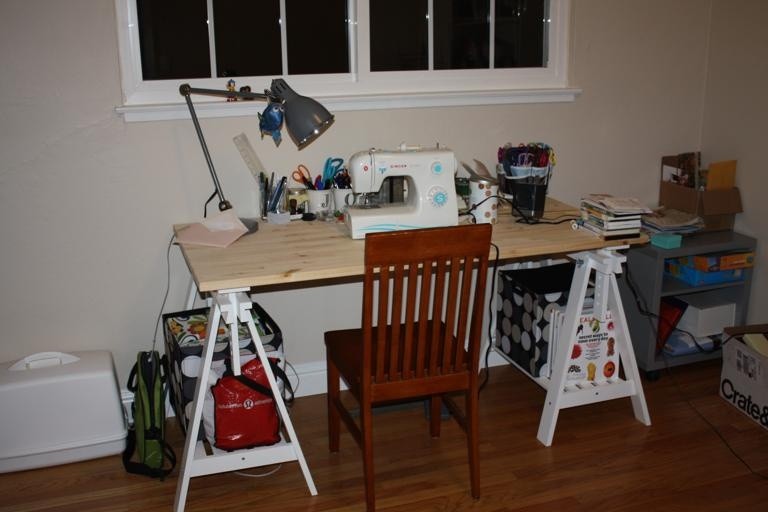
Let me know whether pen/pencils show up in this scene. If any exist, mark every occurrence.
[263,177,269,217]
[318,181,325,189]
[266,172,274,212]
[259,172,263,217]
[269,180,281,216]
[314,175,322,190]
[325,179,330,190]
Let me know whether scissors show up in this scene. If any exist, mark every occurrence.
[505,143,512,149]
[520,143,534,147]
[537,143,549,150]
[497,146,503,166]
[518,153,534,167]
[292,164,316,190]
[331,158,348,179]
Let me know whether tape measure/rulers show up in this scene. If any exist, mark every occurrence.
[232,133,270,188]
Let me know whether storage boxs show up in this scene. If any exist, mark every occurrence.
[1,349,130,479]
[660,181,743,232]
[495,262,597,378]
[161,301,286,441]
[719,323,768,430]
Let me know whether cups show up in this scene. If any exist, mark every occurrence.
[307,188,332,219]
[333,188,353,214]
[260,190,283,220]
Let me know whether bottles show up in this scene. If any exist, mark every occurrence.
[285,189,304,214]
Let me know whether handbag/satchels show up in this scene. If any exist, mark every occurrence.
[122,350,177,482]
[201,350,295,456]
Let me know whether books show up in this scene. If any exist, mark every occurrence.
[578,193,654,238]
[546,305,621,384]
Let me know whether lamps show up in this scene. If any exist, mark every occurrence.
[179,78,335,236]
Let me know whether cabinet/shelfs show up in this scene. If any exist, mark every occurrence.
[616,230,758,372]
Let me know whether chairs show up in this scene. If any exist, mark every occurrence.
[324,223,493,511]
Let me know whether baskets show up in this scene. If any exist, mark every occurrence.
[496,162,551,195]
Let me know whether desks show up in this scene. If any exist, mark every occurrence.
[165,197,651,512]
